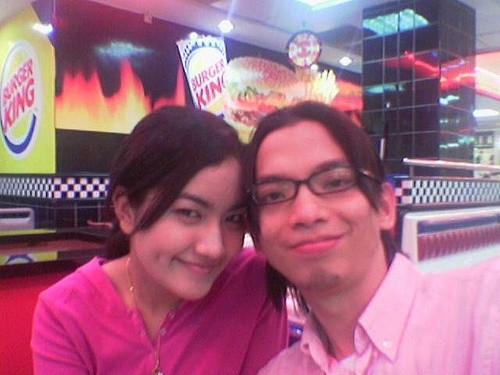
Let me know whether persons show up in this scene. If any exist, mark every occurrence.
[238,100,500,374]
[29,105,290,375]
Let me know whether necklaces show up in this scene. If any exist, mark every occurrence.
[126,256,164,375]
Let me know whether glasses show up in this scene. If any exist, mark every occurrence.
[245,166,384,206]
